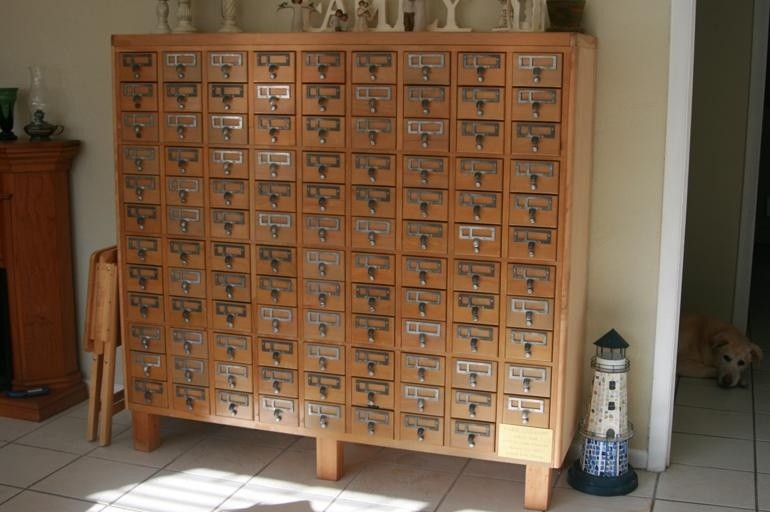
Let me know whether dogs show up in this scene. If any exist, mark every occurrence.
[677,313,763,388]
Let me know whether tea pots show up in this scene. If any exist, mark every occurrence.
[24,109,66,141]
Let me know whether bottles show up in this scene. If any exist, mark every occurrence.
[27,65,52,119]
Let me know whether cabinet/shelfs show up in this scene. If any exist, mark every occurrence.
[1,139,90,423]
[110,31,599,512]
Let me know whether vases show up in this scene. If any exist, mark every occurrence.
[25,65,49,121]
[0,88,19,143]
